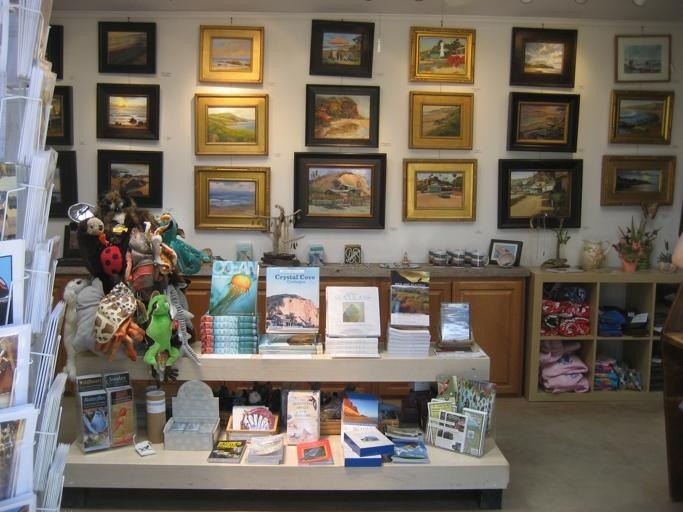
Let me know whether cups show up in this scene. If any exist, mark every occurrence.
[445,248,454,267]
[464,249,472,266]
[433,248,446,267]
[451,248,465,266]
[427,249,434,265]
[471,248,489,267]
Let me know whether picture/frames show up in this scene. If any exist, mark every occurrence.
[409,27,476,83]
[489,239,523,267]
[194,165,271,230]
[608,89,674,145]
[64,226,81,257]
[507,92,580,152]
[601,155,676,206]
[403,159,477,221]
[46,86,73,146]
[99,21,156,73]
[615,35,671,82]
[294,152,386,229]
[310,19,375,78]
[409,91,474,150]
[306,84,380,148]
[98,150,163,208]
[200,25,264,84]
[195,94,269,156]
[46,24,63,78]
[498,159,583,228]
[49,151,78,218]
[97,83,160,140]
[510,27,577,88]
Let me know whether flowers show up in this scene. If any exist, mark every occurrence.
[611,215,663,263]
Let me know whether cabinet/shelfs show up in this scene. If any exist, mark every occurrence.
[661,282,683,502]
[378,264,531,396]
[54,264,378,397]
[0,4,65,512]
[524,267,683,401]
[59,355,510,512]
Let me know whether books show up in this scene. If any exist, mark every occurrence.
[341,426,394,467]
[207,440,246,463]
[200,260,259,356]
[297,438,334,465]
[321,285,380,356]
[260,267,320,355]
[386,270,431,357]
[247,436,284,465]
[342,391,380,440]
[384,425,427,465]
[285,391,318,447]
[0,0,70,511]
[72,370,137,453]
[319,391,344,436]
[426,374,496,458]
[438,303,473,353]
[231,405,274,438]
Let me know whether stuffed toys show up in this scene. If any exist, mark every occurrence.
[60,177,207,388]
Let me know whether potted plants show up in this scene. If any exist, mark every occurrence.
[657,240,672,272]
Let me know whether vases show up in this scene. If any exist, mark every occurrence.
[623,261,636,273]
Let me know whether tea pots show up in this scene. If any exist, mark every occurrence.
[579,237,612,270]
